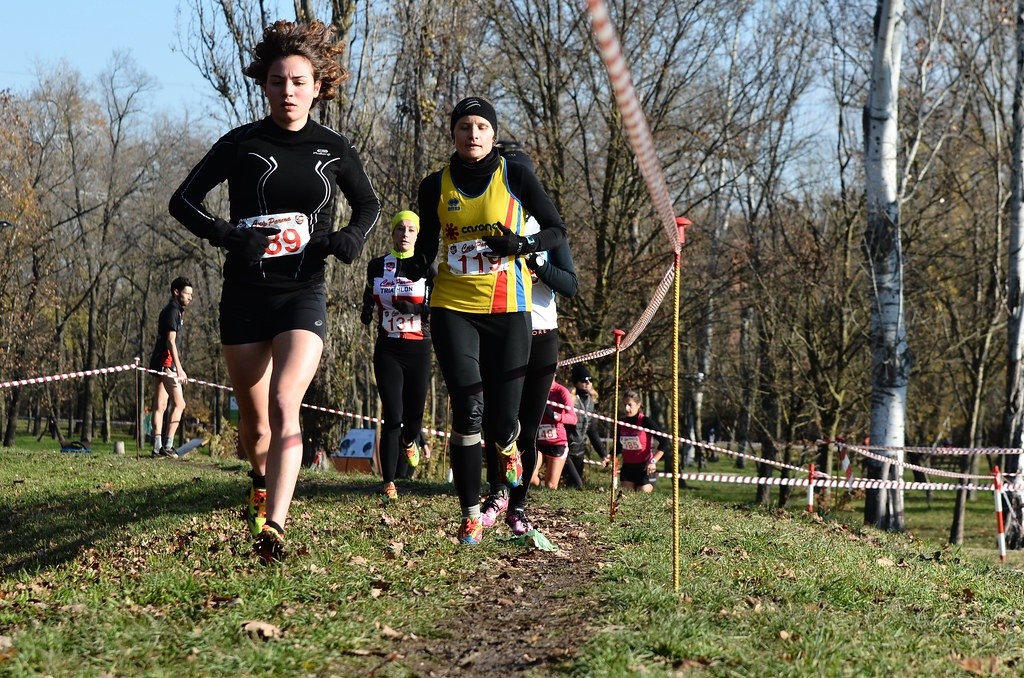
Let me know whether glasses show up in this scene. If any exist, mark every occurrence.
[579,376,593,383]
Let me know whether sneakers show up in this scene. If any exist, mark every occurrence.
[253,524,285,566]
[401,434,420,468]
[383,481,398,500]
[458,513,482,545]
[495,440,523,488]
[151,450,164,460]
[505,507,533,536]
[480,494,509,527]
[247,482,268,538]
[159,446,179,459]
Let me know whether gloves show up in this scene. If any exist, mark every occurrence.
[544,405,555,420]
[326,226,365,264]
[360,305,374,325]
[391,300,421,315]
[481,221,535,258]
[207,218,281,266]
[524,252,546,272]
[404,250,429,282]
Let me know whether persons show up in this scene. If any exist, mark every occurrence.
[480,149,580,536]
[166,19,381,550]
[414,96,571,548]
[396,430,431,480]
[603,391,671,493]
[147,276,194,459]
[360,210,434,501]
[561,364,608,489]
[529,371,578,489]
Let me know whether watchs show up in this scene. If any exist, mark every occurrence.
[650,459,656,464]
[532,255,545,271]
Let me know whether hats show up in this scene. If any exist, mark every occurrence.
[502,150,534,174]
[571,362,592,388]
[391,210,420,236]
[451,97,497,139]
